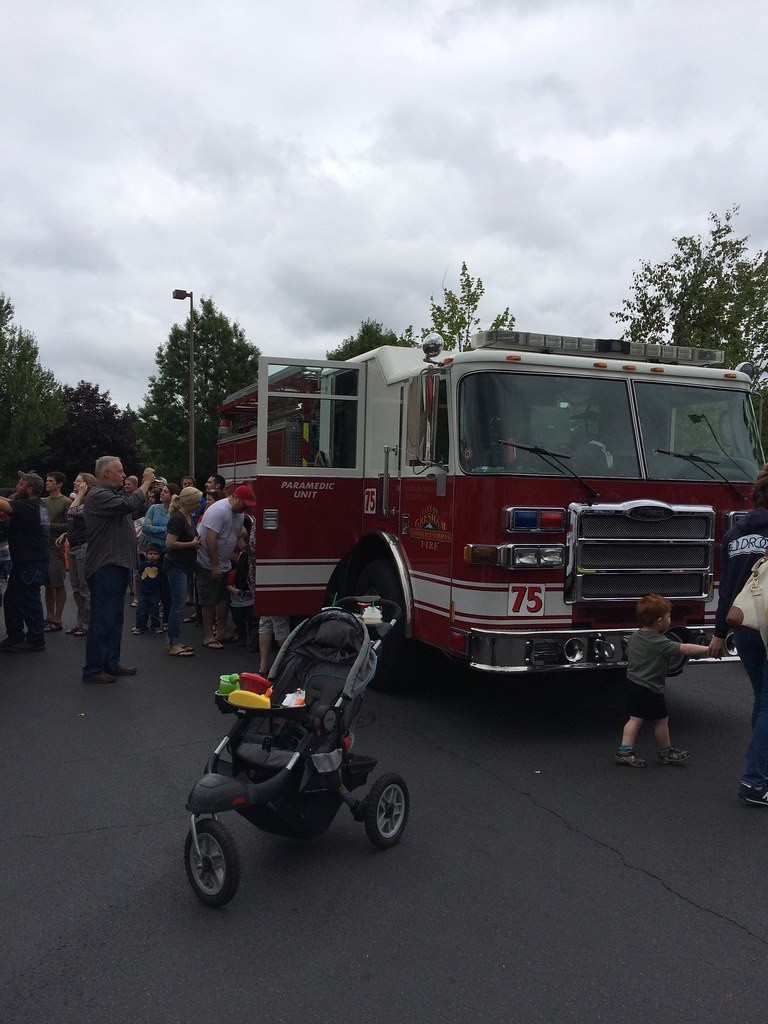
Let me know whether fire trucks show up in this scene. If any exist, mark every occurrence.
[217,331,768,695]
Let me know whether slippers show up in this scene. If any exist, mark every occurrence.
[73,627,88,636]
[42,618,51,627]
[43,621,63,632]
[167,643,195,656]
[65,626,82,634]
[202,640,225,649]
[214,633,240,641]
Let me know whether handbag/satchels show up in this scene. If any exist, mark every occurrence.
[725,550,768,656]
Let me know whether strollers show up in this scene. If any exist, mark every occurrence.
[184,593,411,908]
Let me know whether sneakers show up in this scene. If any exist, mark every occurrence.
[0,630,25,650]
[149,626,163,633]
[133,627,144,634]
[737,779,768,805]
[657,745,691,762]
[612,746,647,768]
[7,632,47,651]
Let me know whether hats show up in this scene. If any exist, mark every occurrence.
[152,475,167,486]
[17,471,44,493]
[233,484,256,506]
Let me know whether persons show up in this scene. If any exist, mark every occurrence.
[614,594,727,768]
[707,463,768,805]
[90,456,156,685]
[118,475,290,675]
[0,469,96,652]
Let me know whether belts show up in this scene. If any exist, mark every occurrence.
[69,540,87,548]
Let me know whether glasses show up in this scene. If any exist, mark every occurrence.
[153,476,162,480]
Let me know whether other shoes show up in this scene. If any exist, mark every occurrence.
[82,672,116,683]
[103,664,137,675]
[181,615,201,623]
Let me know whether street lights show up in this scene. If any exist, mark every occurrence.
[173,289,195,478]
